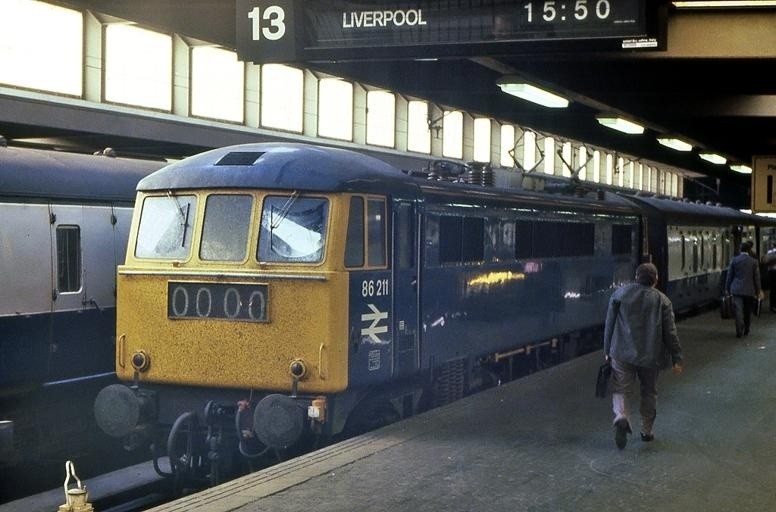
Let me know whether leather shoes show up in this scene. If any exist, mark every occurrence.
[642,433,653,441]
[615,418,628,450]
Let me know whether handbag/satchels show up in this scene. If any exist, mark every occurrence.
[595,363,613,398]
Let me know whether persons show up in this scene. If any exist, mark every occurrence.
[725,240,776,339]
[603,262,683,450]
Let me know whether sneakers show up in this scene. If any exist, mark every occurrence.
[737,328,749,337]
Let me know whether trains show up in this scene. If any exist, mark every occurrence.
[0,135,174,466]
[114,141,776,473]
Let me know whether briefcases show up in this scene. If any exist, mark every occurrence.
[721,295,735,319]
[753,296,761,317]
